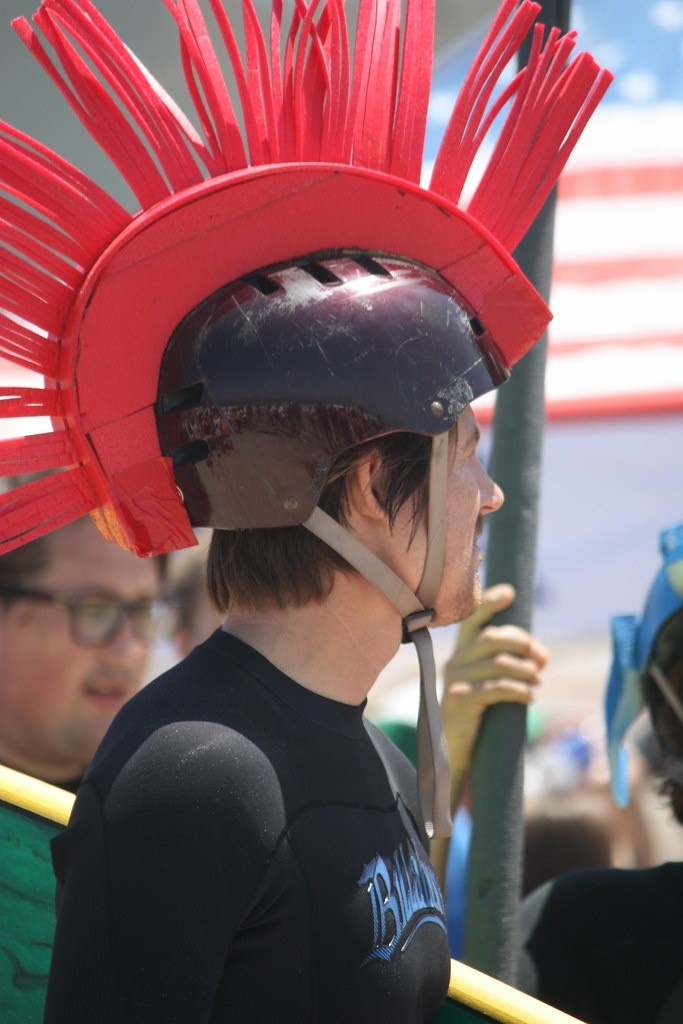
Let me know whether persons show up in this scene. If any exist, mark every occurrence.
[1,441,185,1024]
[161,526,547,962]
[507,543,683,1024]
[45,260,472,1024]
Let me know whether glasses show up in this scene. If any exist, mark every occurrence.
[4,580,178,652]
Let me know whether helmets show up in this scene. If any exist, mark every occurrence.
[0,0,613,557]
